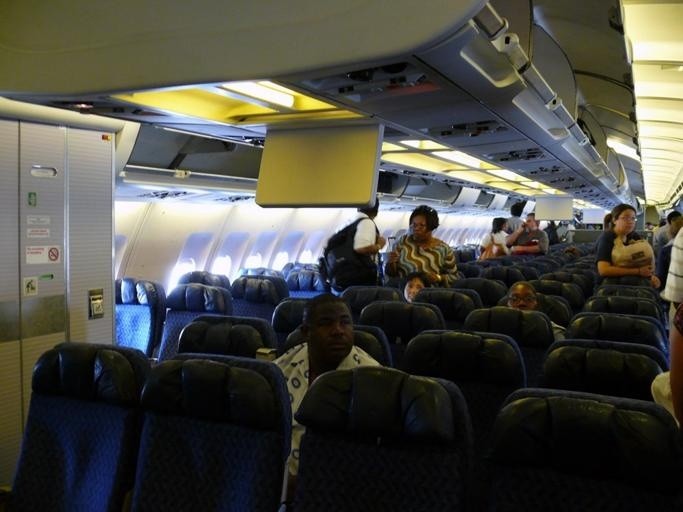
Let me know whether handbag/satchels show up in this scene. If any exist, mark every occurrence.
[480,241,501,262]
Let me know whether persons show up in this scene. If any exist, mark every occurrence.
[594,203,661,289]
[479,218,510,254]
[544,211,613,258]
[385,205,460,288]
[645,210,682,429]
[270,294,385,499]
[335,197,386,292]
[399,270,432,304]
[505,282,567,341]
[507,202,524,235]
[507,213,550,256]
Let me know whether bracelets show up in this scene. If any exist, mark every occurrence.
[638,267,641,275]
[436,274,442,282]
[376,243,382,250]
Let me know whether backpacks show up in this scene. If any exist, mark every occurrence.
[319,216,381,295]
[610,233,656,273]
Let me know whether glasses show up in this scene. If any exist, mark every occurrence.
[507,294,538,307]
[616,214,638,223]
[411,223,427,229]
